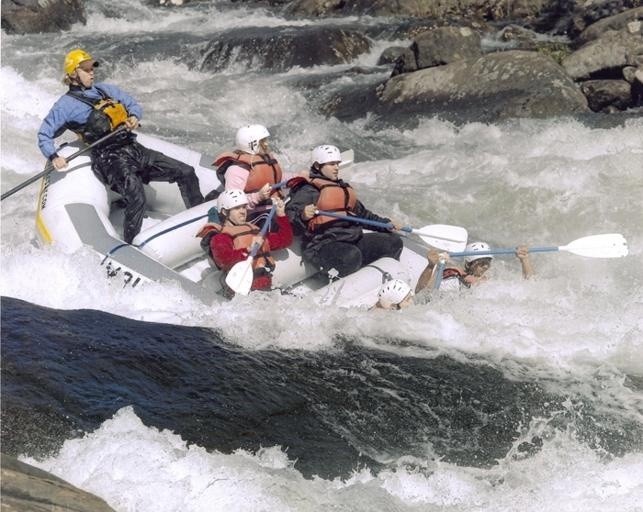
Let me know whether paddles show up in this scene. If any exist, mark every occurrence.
[315,210,468,253]
[269,149,354,190]
[225,197,293,296]
[440,234,629,259]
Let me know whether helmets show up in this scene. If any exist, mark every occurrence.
[378,279,411,310]
[463,243,493,271]
[236,124,271,155]
[311,144,342,168]
[217,188,249,214]
[64,49,92,76]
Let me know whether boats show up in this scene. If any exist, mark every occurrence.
[38,129,442,321]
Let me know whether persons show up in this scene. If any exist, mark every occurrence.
[212,124,285,235]
[195,188,293,301]
[367,279,414,313]
[284,144,403,285]
[37,49,205,244]
[414,241,535,295]
